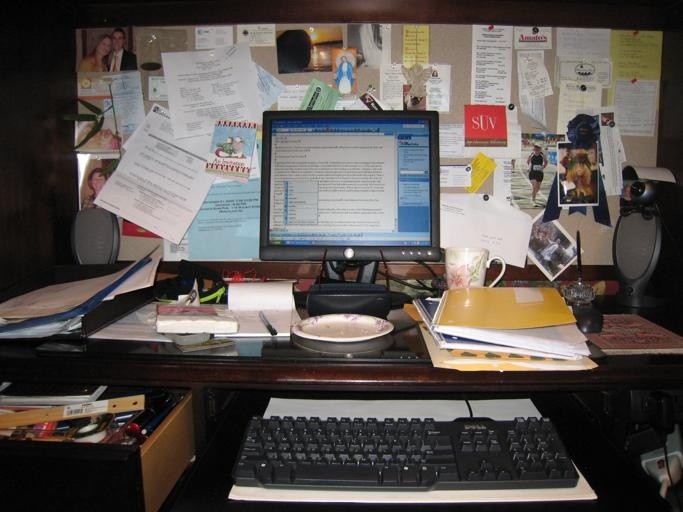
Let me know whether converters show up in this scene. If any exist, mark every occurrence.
[648,393,673,434]
[625,421,665,456]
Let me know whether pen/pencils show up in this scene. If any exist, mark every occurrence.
[126,394,184,435]
[577,231,582,285]
[260,311,277,335]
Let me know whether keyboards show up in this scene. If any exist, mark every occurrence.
[231,417,579,491]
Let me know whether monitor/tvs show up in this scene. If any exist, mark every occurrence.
[260,110,441,310]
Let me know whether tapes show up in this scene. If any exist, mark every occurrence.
[74,424,106,443]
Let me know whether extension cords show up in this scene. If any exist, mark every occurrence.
[641,423,683,486]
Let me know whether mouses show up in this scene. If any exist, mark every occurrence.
[575,309,603,334]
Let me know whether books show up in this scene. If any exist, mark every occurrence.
[156,303,238,334]
[214,281,294,338]
[413,286,592,361]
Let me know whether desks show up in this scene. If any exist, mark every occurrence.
[0,268,682,507]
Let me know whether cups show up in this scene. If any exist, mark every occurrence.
[444,246,505,286]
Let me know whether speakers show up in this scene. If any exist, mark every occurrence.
[612,203,665,309]
[70,208,121,267]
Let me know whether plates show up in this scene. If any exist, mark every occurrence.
[294,332,393,357]
[293,313,395,342]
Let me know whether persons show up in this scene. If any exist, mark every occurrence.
[104,29,137,71]
[537,238,561,264]
[333,57,355,95]
[80,34,111,72]
[82,168,107,208]
[527,146,547,206]
